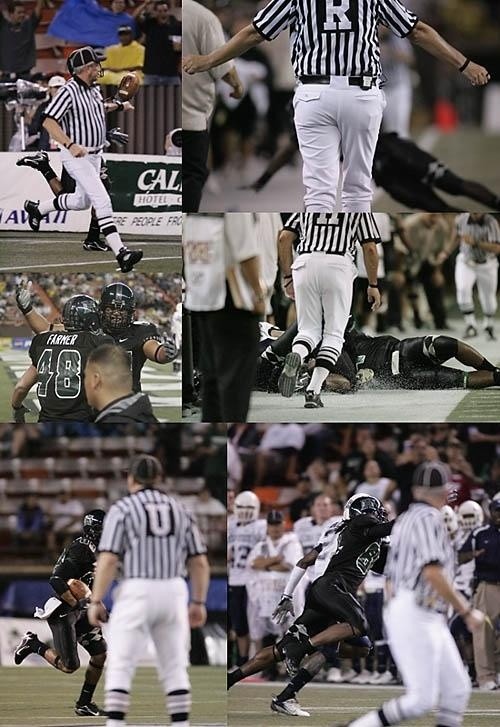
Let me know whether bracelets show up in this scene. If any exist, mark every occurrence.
[66,143,75,149]
[368,284,378,289]
[458,59,470,73]
[284,274,292,279]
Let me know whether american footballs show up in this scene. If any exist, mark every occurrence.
[117,71,140,101]
[69,580,86,600]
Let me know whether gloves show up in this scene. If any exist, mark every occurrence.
[10,404,30,423]
[72,597,90,610]
[271,594,295,624]
[16,279,34,315]
[163,332,179,360]
[107,127,129,148]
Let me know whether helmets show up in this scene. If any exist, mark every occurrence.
[81,509,107,535]
[62,295,101,332]
[99,282,136,330]
[234,491,260,523]
[440,505,458,534]
[349,496,389,522]
[343,493,370,520]
[459,501,484,529]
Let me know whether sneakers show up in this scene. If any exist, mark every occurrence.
[483,325,496,342]
[479,681,498,691]
[24,199,43,232]
[339,668,357,683]
[280,641,301,678]
[74,701,100,716]
[277,352,302,398]
[82,238,109,252]
[115,246,144,272]
[349,669,368,683]
[358,671,379,685]
[13,631,38,665]
[462,326,479,340]
[270,696,311,718]
[326,667,340,682]
[312,668,324,682]
[303,388,324,408]
[15,152,49,170]
[365,669,397,684]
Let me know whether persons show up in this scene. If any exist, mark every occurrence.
[223,441,243,512]
[342,430,398,487]
[131,0,182,84]
[182,0,490,212]
[334,461,493,725]
[12,295,115,424]
[14,509,108,718]
[181,212,265,423]
[1,0,45,83]
[227,497,395,690]
[46,487,84,560]
[460,423,499,450]
[438,212,500,340]
[457,493,500,691]
[294,494,343,557]
[17,46,144,273]
[400,213,451,331]
[344,212,392,337]
[370,22,418,142]
[182,0,244,212]
[95,26,145,86]
[13,279,178,395]
[319,457,360,511]
[87,456,210,727]
[14,495,45,567]
[353,461,401,516]
[443,436,476,478]
[237,96,500,212]
[245,511,304,681]
[27,76,66,151]
[108,1,129,15]
[245,510,304,652]
[256,289,500,392]
[227,491,268,673]
[85,344,161,424]
[258,423,303,486]
[278,212,382,408]
[457,501,484,600]
[395,438,438,510]
[225,422,258,478]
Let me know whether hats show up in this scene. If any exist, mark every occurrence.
[70,46,107,71]
[411,462,449,489]
[47,76,66,88]
[266,510,283,524]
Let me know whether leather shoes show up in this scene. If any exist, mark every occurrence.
[182,400,202,417]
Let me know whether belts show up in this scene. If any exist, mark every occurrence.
[298,75,377,87]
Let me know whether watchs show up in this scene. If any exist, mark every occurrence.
[460,601,474,617]
[190,598,208,606]
[88,599,105,604]
